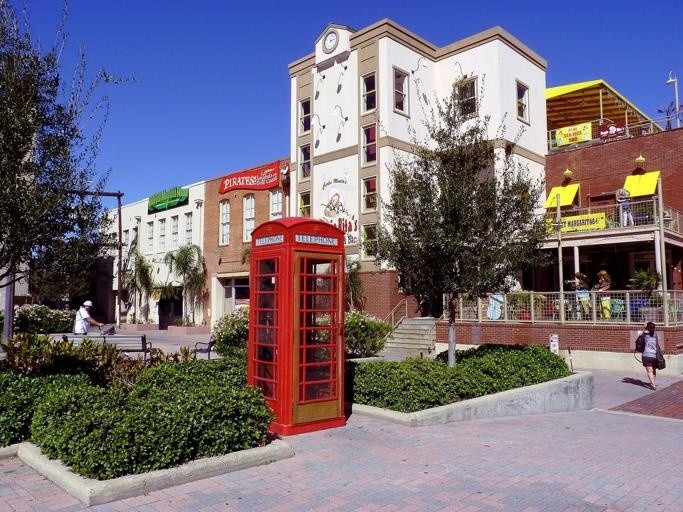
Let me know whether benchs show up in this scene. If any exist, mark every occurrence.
[62,334,156,365]
[192,335,215,360]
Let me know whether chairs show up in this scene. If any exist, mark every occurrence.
[552,295,624,323]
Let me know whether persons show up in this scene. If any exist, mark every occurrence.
[70,299,105,336]
[567,271,591,321]
[589,268,612,322]
[633,321,666,392]
[614,188,635,228]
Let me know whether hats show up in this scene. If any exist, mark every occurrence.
[83,300,93,307]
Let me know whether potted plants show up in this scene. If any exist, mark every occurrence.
[508,293,531,322]
[632,270,662,324]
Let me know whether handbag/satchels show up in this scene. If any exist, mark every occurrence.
[654,333,666,370]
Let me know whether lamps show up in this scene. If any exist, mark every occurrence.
[454,61,467,79]
[330,105,348,121]
[635,154,645,171]
[331,56,347,71]
[310,65,326,81]
[309,114,326,130]
[561,165,572,178]
[411,57,428,74]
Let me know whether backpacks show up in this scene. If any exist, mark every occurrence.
[635,334,645,353]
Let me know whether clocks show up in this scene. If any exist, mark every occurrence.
[321,28,338,54]
